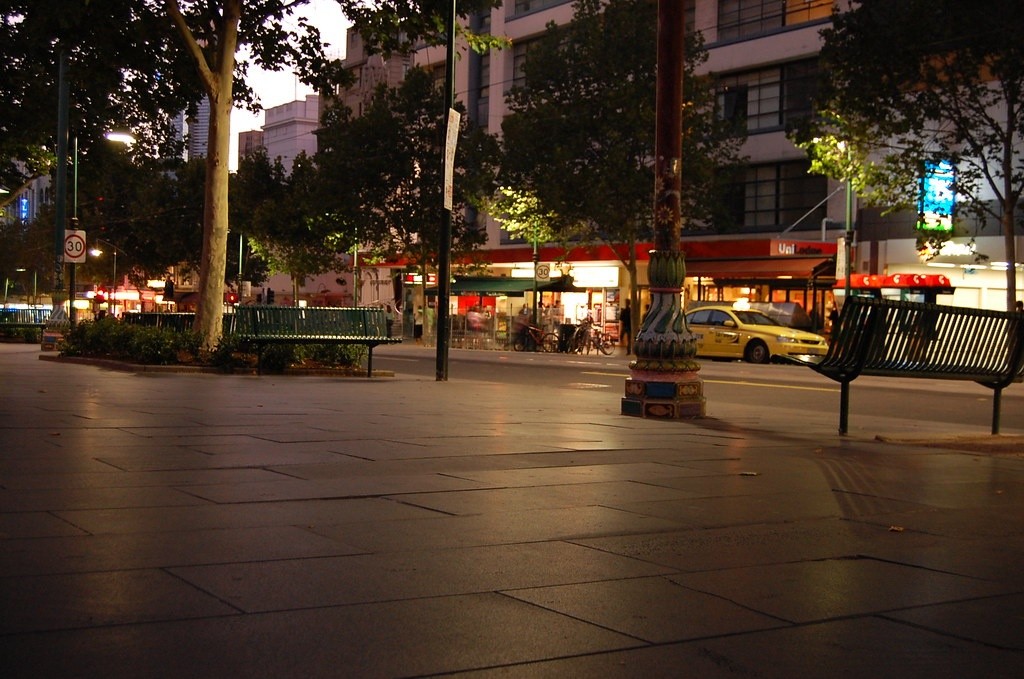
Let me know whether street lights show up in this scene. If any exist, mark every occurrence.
[92,238,128,317]
[815,135,852,303]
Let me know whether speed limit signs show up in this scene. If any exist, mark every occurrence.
[64,230,86,263]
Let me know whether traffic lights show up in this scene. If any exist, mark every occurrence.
[267,288,274,305]
[228,293,236,302]
[257,293,262,301]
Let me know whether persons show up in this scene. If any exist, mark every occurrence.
[1015,301,1024,313]
[618,297,632,357]
[378,303,434,344]
[517,299,563,325]
[641,302,649,324]
[579,310,594,356]
[448,305,492,341]
[809,301,843,343]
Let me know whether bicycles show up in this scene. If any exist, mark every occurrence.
[513,323,561,353]
[565,319,616,357]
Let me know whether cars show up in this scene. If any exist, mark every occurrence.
[684,305,829,365]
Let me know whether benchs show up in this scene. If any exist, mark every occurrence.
[232,305,402,377]
[0,307,52,343]
[770,295,1024,437]
[122,311,194,333]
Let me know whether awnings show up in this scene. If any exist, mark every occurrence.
[424,277,564,297]
[683,258,836,279]
